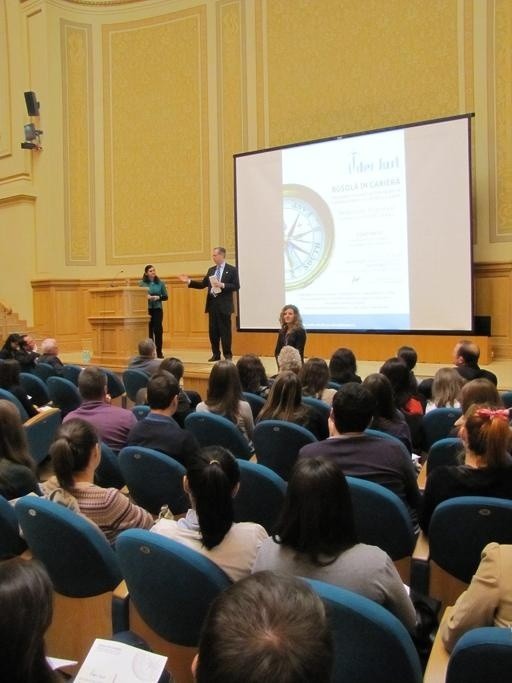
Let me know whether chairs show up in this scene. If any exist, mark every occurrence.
[1,361,512,680]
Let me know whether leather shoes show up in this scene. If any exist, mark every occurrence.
[208,354,220,361]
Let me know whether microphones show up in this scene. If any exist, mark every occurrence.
[110,271,123,287]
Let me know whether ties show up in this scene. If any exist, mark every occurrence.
[212,266,221,298]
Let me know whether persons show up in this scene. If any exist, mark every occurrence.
[138,264,168,359]
[274,304,307,372]
[177,247,241,362]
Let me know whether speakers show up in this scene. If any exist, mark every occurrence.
[24,92,40,116]
